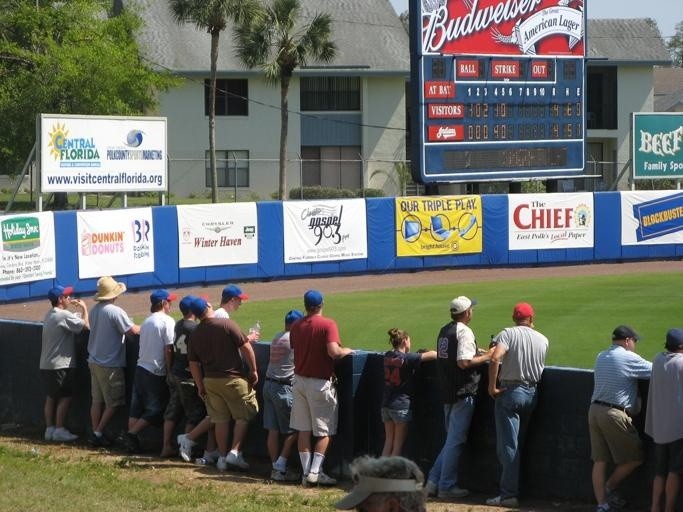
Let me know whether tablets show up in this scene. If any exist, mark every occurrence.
[405,220,419,238]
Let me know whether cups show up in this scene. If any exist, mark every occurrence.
[248,327,255,341]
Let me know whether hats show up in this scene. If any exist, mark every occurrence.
[665,329,682,345]
[284,310,303,324]
[222,285,249,299]
[303,290,321,306]
[179,294,208,315]
[333,475,422,510]
[513,303,533,319]
[48,285,72,301]
[612,325,641,339]
[449,296,476,315]
[150,290,176,302]
[94,276,126,301]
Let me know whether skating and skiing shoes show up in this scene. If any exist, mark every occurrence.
[44,427,143,454]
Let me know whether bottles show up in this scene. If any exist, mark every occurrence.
[253,320,260,342]
[488,334,495,349]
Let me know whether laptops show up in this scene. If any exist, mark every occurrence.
[431,216,450,238]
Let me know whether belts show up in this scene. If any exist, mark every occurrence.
[265,378,290,385]
[593,400,623,411]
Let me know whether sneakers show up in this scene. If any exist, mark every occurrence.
[485,496,518,509]
[423,487,469,498]
[270,469,336,487]
[596,493,625,512]
[177,434,248,471]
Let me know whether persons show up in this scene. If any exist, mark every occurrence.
[160,294,201,458]
[178,281,260,465]
[88,276,141,446]
[486,302,549,508]
[127,289,177,454]
[644,329,683,512]
[187,294,258,471]
[588,325,652,511]
[425,296,500,499]
[38,286,88,441]
[380,328,437,455]
[289,290,355,487]
[263,310,304,480]
